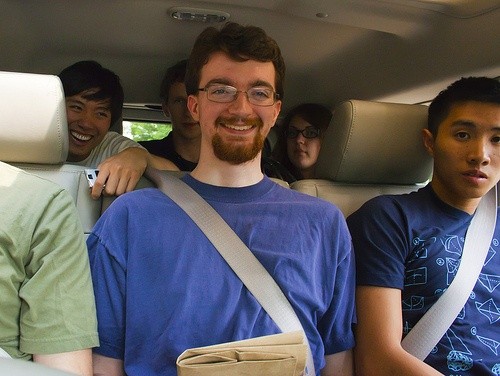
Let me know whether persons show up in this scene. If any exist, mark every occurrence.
[85,22,356,376]
[136,60,203,172]
[344,77,500,376]
[57,60,180,200]
[0,160,100,376]
[271,104,333,181]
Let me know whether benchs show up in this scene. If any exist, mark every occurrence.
[0,71,434,240]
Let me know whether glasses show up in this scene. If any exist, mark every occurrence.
[198,81,280,106]
[286,125,320,138]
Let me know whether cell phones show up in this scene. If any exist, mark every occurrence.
[84,168,107,189]
